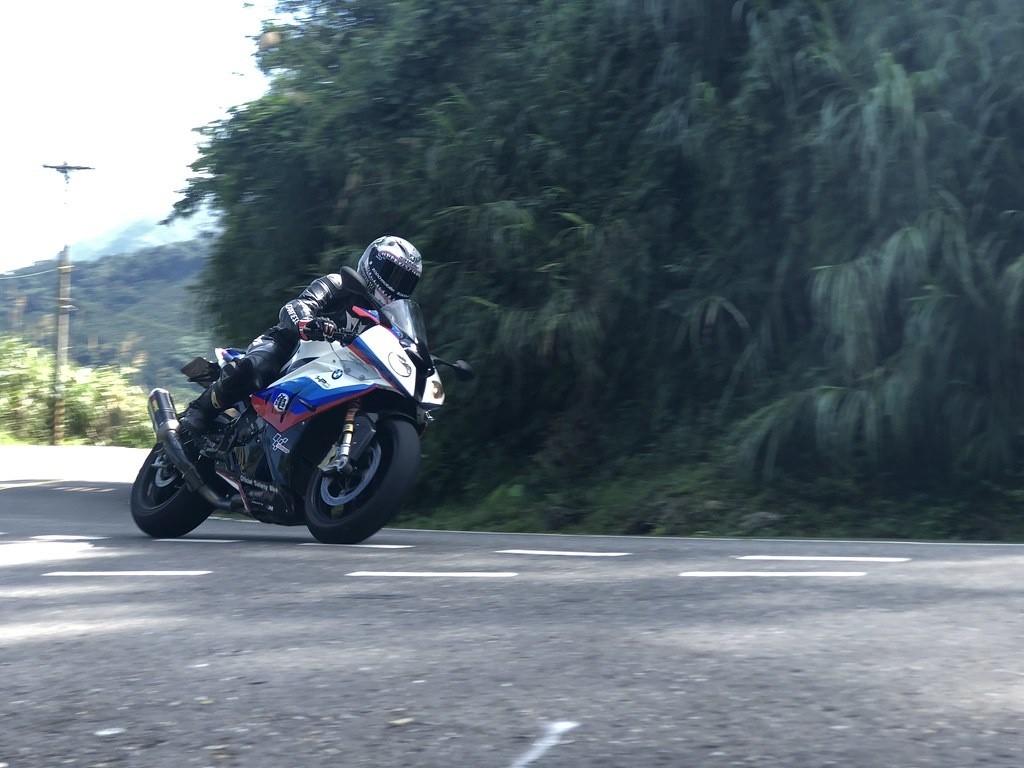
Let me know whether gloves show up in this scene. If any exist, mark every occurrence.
[303,316,338,343]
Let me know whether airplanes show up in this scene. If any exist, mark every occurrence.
[42,160,97,181]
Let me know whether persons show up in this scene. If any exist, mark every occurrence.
[182,235,423,438]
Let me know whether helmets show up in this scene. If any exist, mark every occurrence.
[357,235,422,309]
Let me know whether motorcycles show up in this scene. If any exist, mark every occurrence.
[129,296,476,546]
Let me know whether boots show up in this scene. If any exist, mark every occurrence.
[178,382,234,438]
